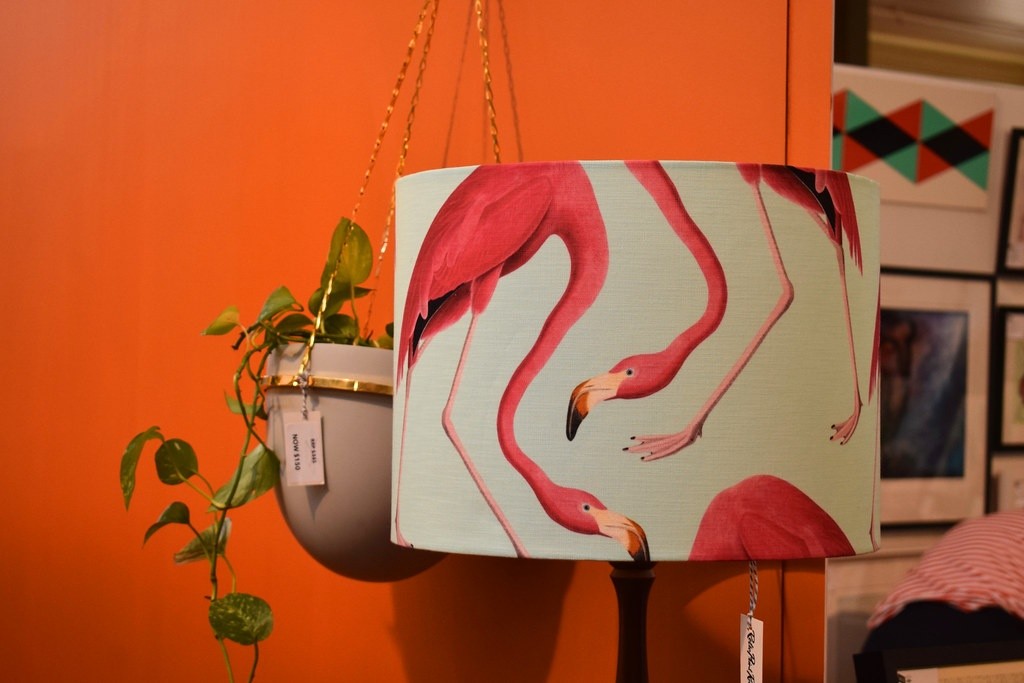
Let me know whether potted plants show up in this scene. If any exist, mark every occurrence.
[118,216,449,683]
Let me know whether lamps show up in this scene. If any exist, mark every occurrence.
[389,161,881,682]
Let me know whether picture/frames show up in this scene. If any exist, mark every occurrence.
[832,75,999,212]
[996,129,1024,278]
[880,264,996,531]
[992,307,1024,453]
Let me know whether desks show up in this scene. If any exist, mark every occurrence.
[896,661,1024,683]
